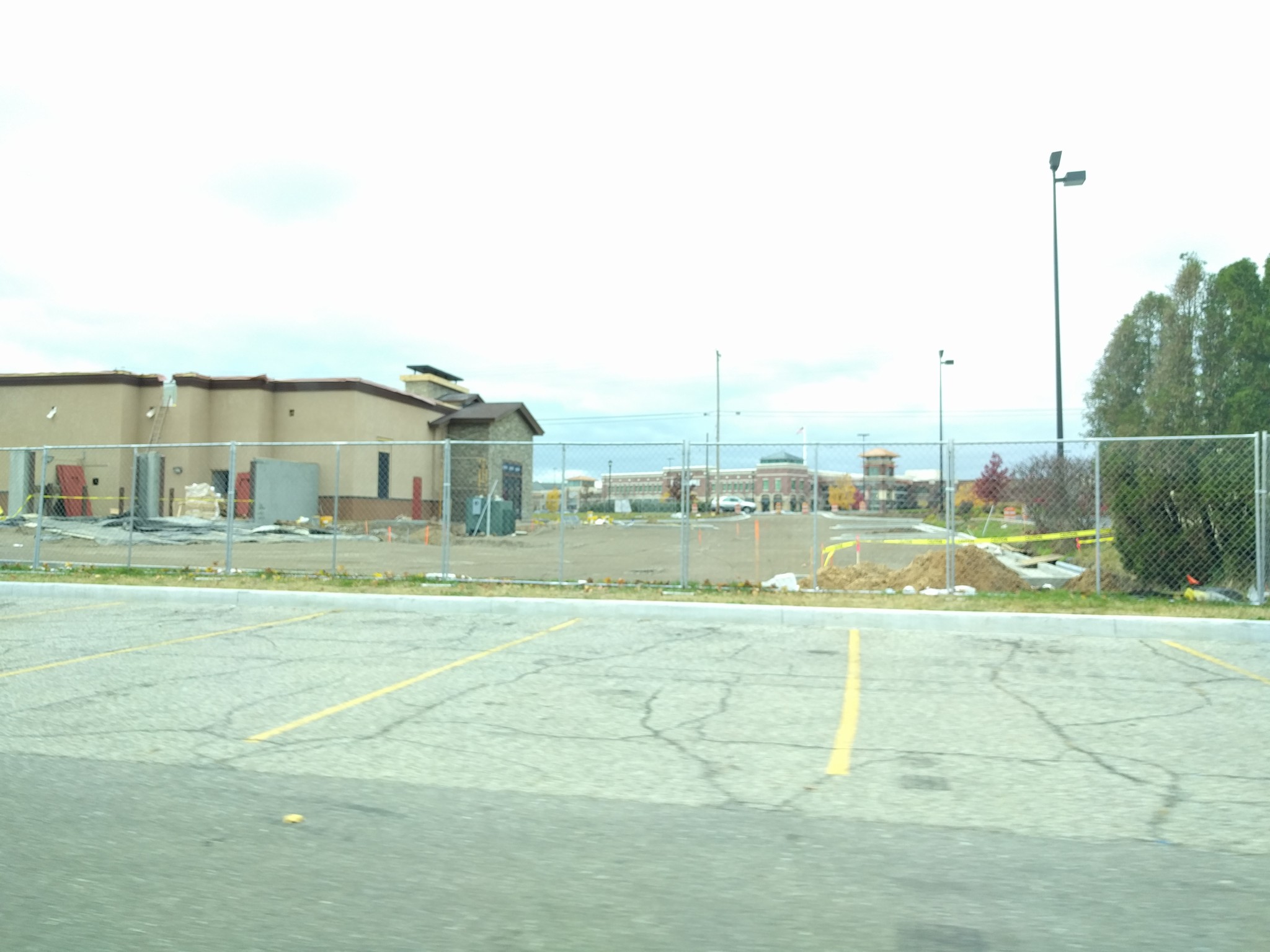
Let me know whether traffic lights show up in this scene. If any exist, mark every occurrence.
[939,350,953,511]
[1050,150,1085,518]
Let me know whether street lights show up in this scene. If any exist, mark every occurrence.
[715,351,721,516]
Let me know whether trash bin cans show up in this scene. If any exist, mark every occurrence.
[488,498,515,535]
[465,495,490,535]
